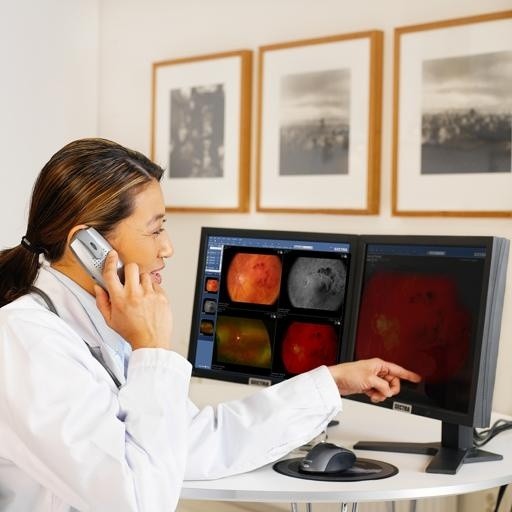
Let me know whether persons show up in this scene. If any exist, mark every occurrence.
[0,138,421,512]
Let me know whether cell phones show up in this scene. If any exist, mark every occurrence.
[68,225,125,300]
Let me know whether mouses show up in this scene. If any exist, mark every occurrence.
[298,442,356,473]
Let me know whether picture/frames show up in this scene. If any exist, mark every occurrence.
[387,9,510,218]
[254,28,388,218]
[150,48,253,216]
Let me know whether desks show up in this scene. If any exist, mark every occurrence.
[167,396,512,512]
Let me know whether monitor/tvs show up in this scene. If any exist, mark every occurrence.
[185,225,358,388]
[340,234,510,475]
